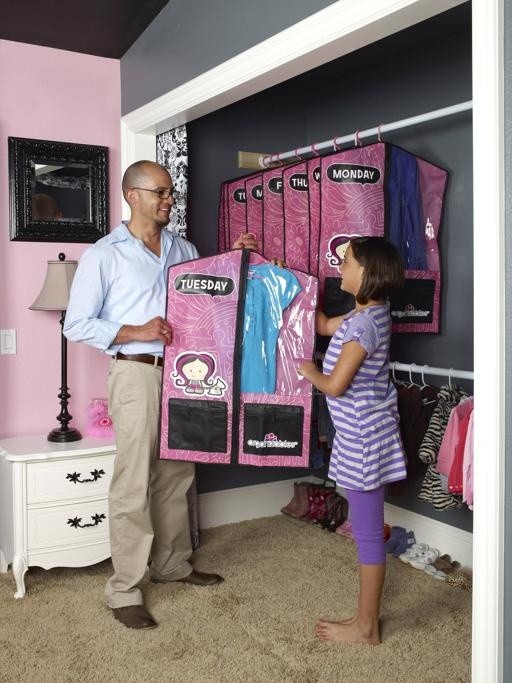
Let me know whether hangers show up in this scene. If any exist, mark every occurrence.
[268,123,384,167]
[240,230,268,280]
[390,359,455,397]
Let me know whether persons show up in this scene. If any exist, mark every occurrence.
[270,235,407,647]
[62,160,258,631]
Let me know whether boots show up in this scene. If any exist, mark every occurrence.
[385,526,405,553]
[392,530,416,557]
[281,481,348,532]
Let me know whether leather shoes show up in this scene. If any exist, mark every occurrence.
[151,568,221,585]
[113,605,158,630]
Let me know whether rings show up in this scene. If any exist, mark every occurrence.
[162,329,169,335]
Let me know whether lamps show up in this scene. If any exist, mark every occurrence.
[28,251,84,444]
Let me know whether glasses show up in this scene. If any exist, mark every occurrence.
[131,188,179,199]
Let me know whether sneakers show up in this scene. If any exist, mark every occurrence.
[398,543,460,580]
[336,520,352,538]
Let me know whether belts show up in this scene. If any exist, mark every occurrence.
[112,353,163,366]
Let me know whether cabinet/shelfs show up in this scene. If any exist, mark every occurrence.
[1,432,123,600]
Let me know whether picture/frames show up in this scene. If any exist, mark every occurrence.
[7,136,110,244]
